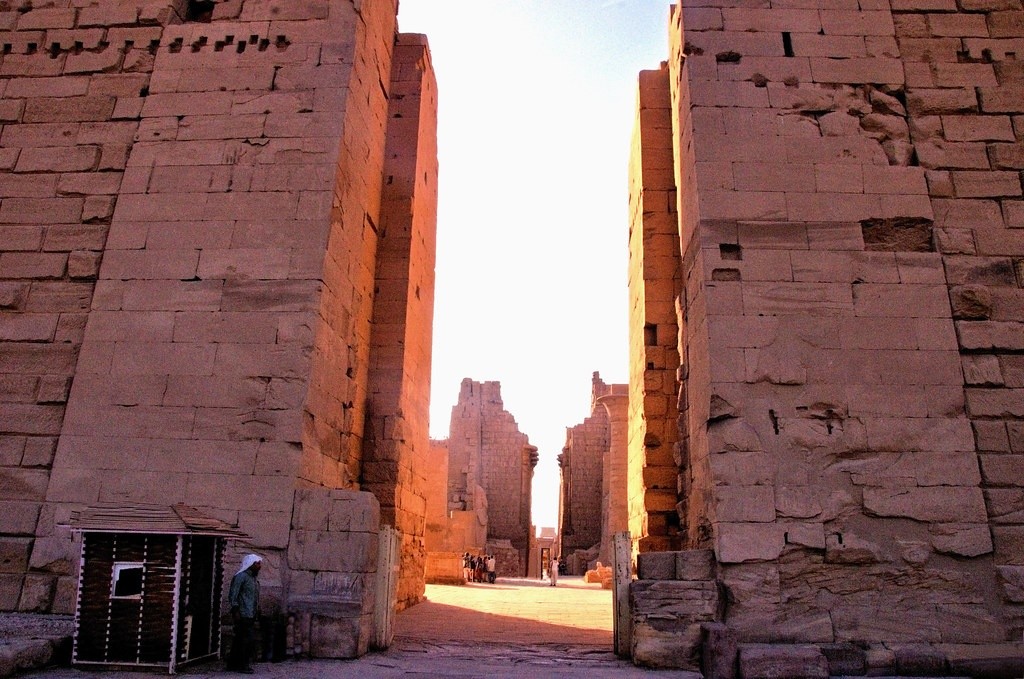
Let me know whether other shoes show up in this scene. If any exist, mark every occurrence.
[234,664,253,674]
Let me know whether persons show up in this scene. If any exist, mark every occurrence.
[549,557,587,586]
[462,552,497,584]
[229,554,265,673]
